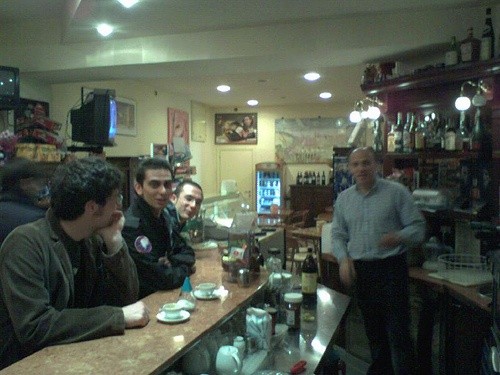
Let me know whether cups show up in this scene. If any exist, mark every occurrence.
[195,283,217,296]
[237,269,251,288]
[265,307,278,335]
[162,303,185,319]
[284,292,303,332]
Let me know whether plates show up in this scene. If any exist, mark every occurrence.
[155,310,190,324]
[194,290,220,299]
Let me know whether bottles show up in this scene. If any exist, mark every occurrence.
[373,106,486,153]
[300,248,318,306]
[300,303,318,351]
[265,247,283,304]
[259,171,278,205]
[296,171,325,186]
[445,8,494,68]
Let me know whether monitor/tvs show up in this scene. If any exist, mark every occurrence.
[0,65,20,103]
[71,94,117,145]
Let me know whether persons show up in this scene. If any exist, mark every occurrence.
[236,115,256,139]
[0,158,149,371]
[331,146,426,375]
[164,181,203,236]
[121,158,196,302]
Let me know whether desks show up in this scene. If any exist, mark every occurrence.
[290,227,321,272]
[409,264,495,375]
[257,208,294,225]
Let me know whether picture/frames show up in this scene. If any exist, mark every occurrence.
[215,112,257,145]
[7,93,55,137]
[115,97,137,137]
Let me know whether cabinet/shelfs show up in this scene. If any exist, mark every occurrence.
[360,57,500,232]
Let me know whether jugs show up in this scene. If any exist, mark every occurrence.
[181,328,244,375]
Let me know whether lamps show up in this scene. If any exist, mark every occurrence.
[347,95,383,123]
[454,77,494,111]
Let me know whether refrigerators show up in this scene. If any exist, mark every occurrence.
[254,162,287,230]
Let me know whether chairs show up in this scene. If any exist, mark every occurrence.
[286,208,321,274]
